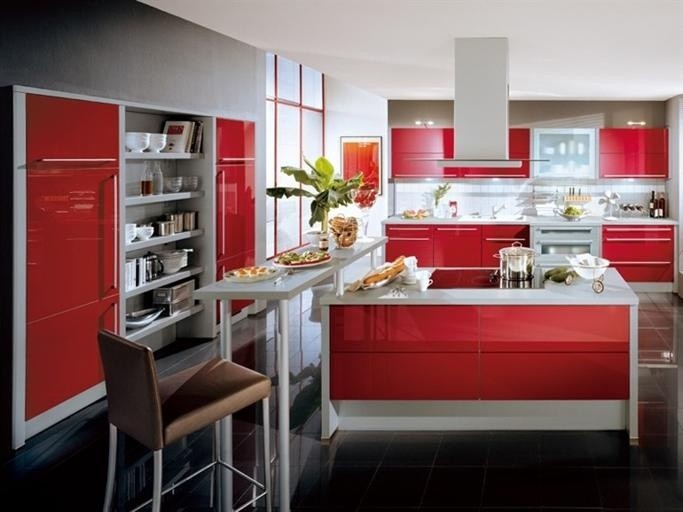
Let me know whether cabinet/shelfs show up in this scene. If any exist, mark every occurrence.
[215,118,257,162]
[0,295,121,421]
[391,127,458,177]
[213,163,256,261]
[484,225,530,269]
[432,226,483,268]
[385,239,434,267]
[217,250,255,328]
[0,175,120,325]
[119,100,217,342]
[458,129,530,176]
[529,129,597,177]
[598,128,670,176]
[385,224,434,238]
[603,226,674,283]
[0,93,120,176]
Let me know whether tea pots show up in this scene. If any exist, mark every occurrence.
[141,251,163,280]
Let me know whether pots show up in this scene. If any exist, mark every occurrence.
[493,241,537,280]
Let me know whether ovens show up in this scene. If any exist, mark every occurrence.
[530,222,603,266]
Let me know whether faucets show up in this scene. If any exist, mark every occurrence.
[492,204,507,218]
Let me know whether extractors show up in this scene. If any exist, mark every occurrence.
[436,35,524,169]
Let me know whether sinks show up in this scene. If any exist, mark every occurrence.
[457,213,527,222]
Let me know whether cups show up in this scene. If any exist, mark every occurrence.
[415,276,432,292]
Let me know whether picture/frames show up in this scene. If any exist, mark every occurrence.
[341,136,382,195]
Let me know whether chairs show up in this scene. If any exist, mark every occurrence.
[98,327,273,508]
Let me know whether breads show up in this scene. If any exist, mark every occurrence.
[363,255,404,285]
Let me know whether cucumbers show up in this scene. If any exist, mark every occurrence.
[545,268,576,283]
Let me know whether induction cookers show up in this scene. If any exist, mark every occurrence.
[427,269,544,289]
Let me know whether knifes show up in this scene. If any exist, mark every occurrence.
[568,187,581,196]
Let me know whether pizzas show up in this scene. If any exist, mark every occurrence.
[277,251,329,264]
[233,266,268,277]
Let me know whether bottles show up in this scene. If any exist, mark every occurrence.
[152,159,164,195]
[560,139,583,155]
[620,203,644,212]
[140,159,152,195]
[449,200,456,217]
[649,189,667,218]
[319,236,328,250]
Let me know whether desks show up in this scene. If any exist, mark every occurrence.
[192,236,387,470]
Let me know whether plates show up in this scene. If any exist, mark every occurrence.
[273,251,332,268]
[401,282,417,285]
[124,307,168,330]
[223,266,277,283]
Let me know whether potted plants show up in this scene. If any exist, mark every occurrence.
[268,156,362,250]
[432,181,451,221]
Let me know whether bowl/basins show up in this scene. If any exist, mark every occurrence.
[164,176,199,192]
[305,230,327,247]
[125,131,168,153]
[125,223,155,244]
[163,250,187,274]
